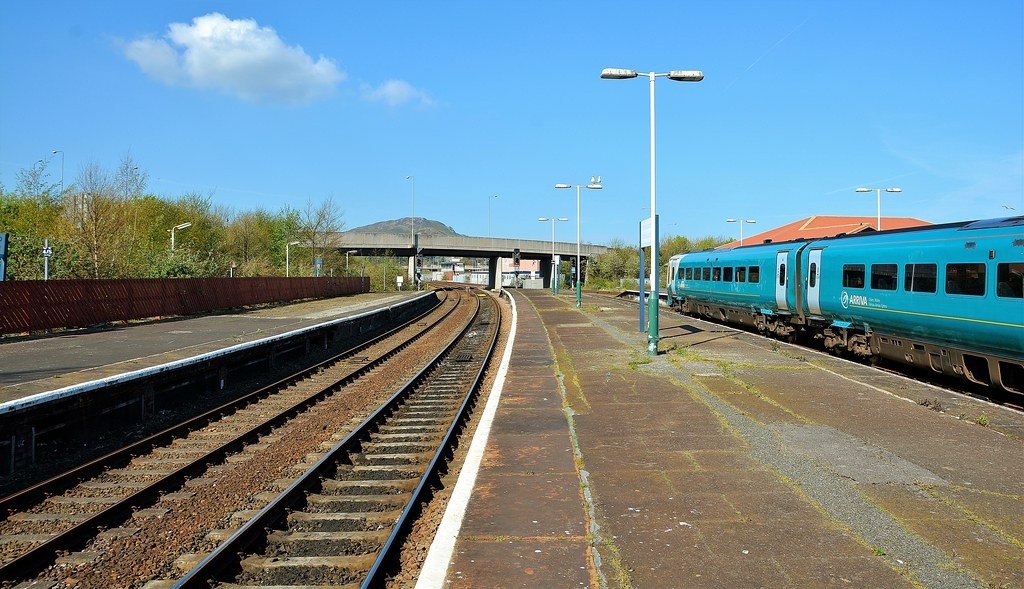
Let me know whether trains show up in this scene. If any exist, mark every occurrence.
[666,216,1024,401]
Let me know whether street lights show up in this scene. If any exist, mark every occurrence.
[34,160,42,198]
[346,250,357,277]
[126,165,138,199]
[857,187,902,230]
[725,218,756,247]
[53,151,64,195]
[172,222,192,253]
[286,241,300,277]
[406,175,414,243]
[537,218,568,296]
[600,68,704,356]
[555,184,602,308]
[489,195,497,238]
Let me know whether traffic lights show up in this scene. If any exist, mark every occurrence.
[514,255,520,266]
[416,258,422,268]
[570,257,576,268]
